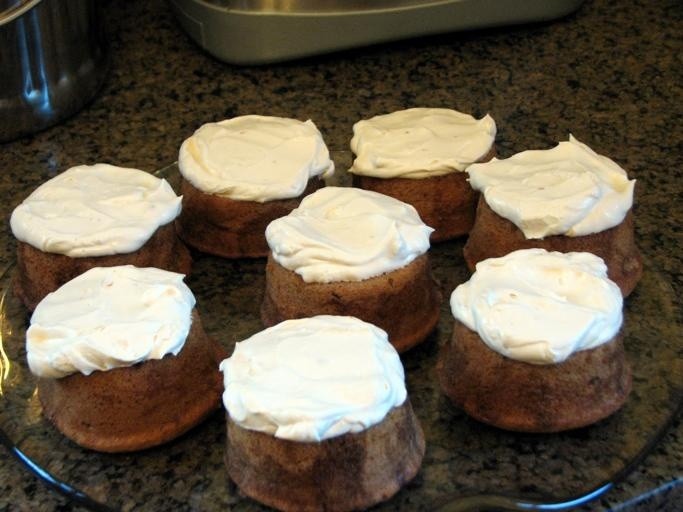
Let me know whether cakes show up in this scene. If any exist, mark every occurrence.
[347,106,497,244]
[435,246,633,434]
[218,314,426,512]
[260,185,442,355]
[10,162,195,312]
[462,131,644,302]
[175,114,336,260]
[25,263,227,455]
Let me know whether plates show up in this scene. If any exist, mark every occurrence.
[1,142,682,511]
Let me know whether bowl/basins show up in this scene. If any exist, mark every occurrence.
[1,1,115,144]
